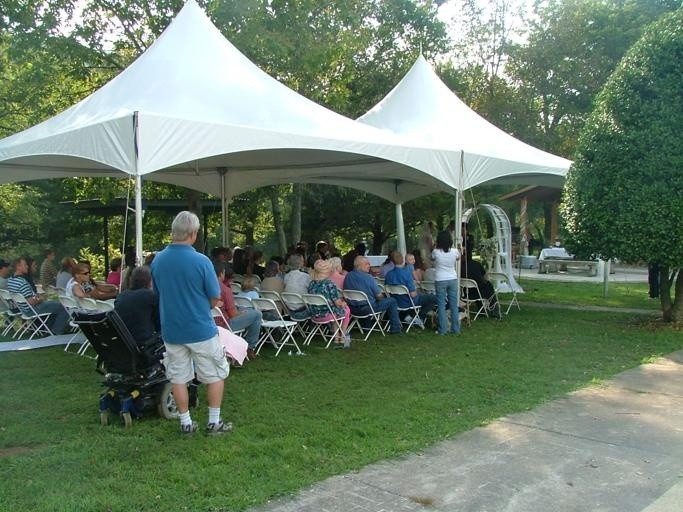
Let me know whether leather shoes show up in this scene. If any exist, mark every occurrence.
[247,349,256,359]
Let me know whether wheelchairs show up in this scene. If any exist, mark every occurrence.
[68,310,199,427]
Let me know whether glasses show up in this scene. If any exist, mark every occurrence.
[79,270,91,275]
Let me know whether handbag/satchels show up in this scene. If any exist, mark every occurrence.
[93,283,118,300]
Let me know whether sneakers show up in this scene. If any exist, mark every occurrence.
[179,421,198,432]
[206,421,234,435]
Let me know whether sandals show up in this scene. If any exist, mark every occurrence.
[334,337,339,343]
[339,337,351,344]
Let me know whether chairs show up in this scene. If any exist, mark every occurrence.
[0,273,524,356]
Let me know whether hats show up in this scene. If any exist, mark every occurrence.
[0,259,11,266]
[309,259,333,281]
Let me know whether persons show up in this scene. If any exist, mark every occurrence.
[151,210,234,439]
[113,265,162,352]
[210,239,437,360]
[0,249,137,338]
[418,221,499,335]
